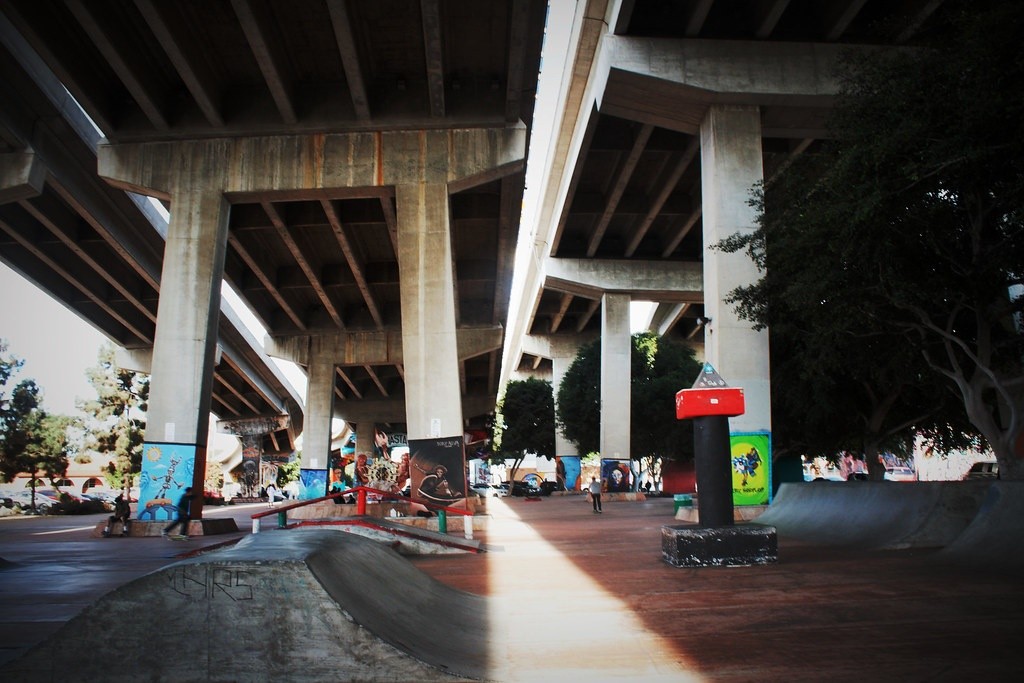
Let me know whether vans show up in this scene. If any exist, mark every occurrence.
[501,481,540,497]
[472,484,508,497]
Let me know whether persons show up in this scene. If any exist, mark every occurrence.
[645,480,651,494]
[101,494,131,537]
[638,480,643,492]
[587,476,602,512]
[162,486,197,539]
[266,484,275,508]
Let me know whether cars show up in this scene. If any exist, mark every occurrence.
[0,489,138,510]
